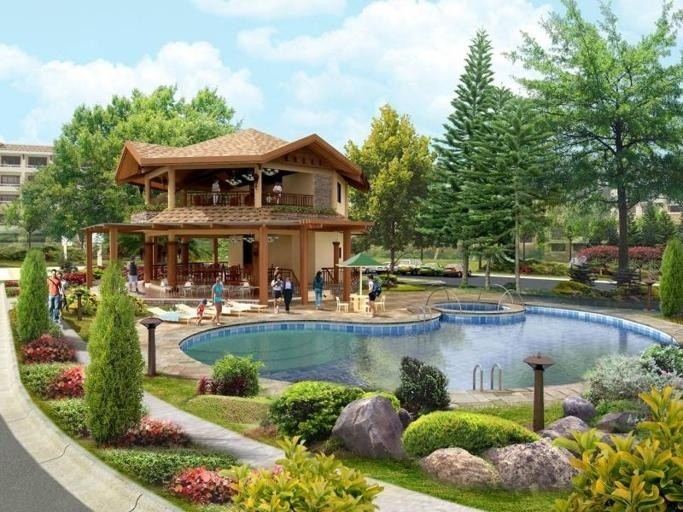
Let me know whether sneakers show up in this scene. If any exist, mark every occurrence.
[274,306,280,314]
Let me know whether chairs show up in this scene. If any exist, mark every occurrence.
[206,301,268,317]
[175,303,216,320]
[156,260,259,300]
[336,293,385,312]
[148,307,196,326]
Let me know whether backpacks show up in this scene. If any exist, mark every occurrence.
[372,281,382,296]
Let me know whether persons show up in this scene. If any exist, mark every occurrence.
[195,299,212,326]
[126,257,138,293]
[312,271,324,310]
[570,251,594,286]
[367,270,382,317]
[212,277,223,325]
[266,182,282,204]
[47,270,67,322]
[270,272,293,313]
[212,180,219,206]
